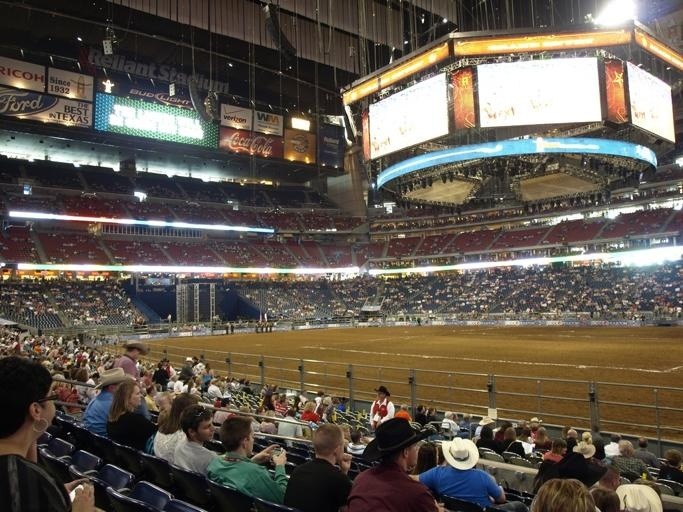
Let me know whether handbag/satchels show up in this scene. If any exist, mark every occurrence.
[145,433,156,455]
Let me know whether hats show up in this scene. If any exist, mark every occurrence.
[616,484,663,512]
[539,452,608,487]
[479,416,494,426]
[186,357,194,363]
[375,386,390,396]
[361,416,432,462]
[441,437,479,470]
[529,417,542,423]
[95,368,136,389]
[123,340,147,354]
[573,441,596,458]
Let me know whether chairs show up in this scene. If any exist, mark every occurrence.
[0,280,337,329]
[38,391,683,512]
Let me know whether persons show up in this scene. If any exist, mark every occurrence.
[0,159,683,334]
[0,328,683,512]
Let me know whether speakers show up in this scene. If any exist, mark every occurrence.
[261,4,296,60]
[187,78,209,122]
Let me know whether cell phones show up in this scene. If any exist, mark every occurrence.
[270,447,280,463]
